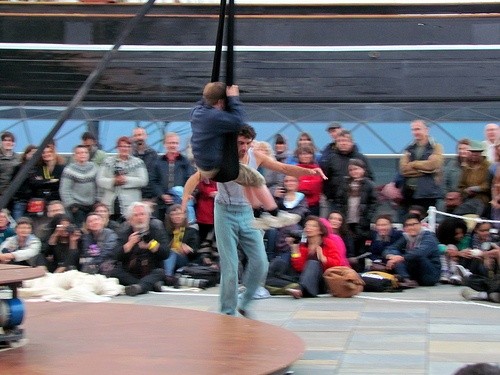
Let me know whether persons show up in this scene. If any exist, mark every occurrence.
[190,81,300,230]
[0,120,499,302]
[180,124,329,319]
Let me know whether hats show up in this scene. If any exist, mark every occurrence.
[468,142,484,152]
[326,122,341,131]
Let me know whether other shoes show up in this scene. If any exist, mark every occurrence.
[154,281,164,290]
[461,287,486,300]
[439,276,448,283]
[125,284,140,294]
[451,274,462,284]
[398,278,416,287]
[287,287,303,298]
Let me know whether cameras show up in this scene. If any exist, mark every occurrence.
[137,228,153,243]
